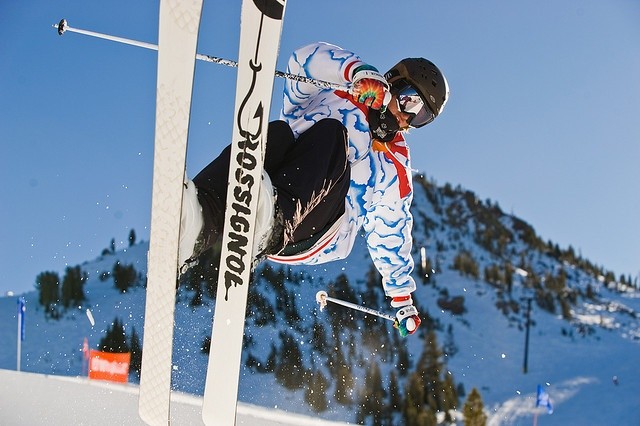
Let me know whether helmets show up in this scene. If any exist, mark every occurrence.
[385,59,448,128]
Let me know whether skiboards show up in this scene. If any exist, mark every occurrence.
[138,0,287,426]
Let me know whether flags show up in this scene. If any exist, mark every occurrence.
[537,383,553,415]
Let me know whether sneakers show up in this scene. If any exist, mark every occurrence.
[180,178,203,273]
[252,171,277,273]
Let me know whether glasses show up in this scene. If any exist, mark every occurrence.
[395,85,434,128]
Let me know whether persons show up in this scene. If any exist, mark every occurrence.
[177,42,449,337]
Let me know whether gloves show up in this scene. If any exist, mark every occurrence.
[349,67,391,108]
[391,298,422,336]
